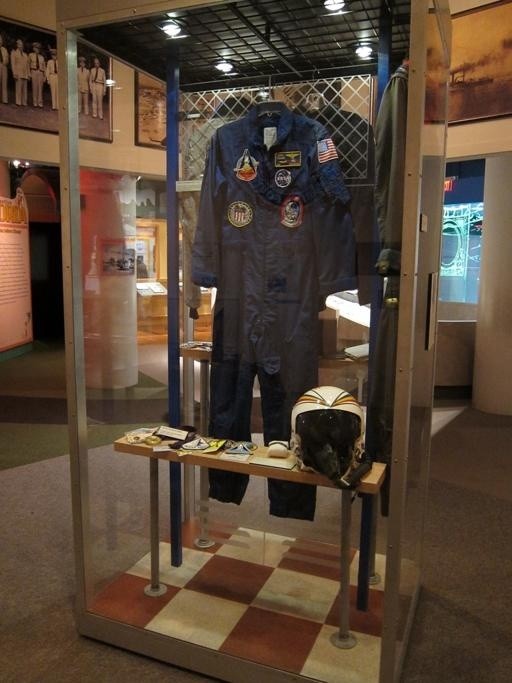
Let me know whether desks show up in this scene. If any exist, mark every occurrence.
[180,341,370,520]
[114,426,386,647]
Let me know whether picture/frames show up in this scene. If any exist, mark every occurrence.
[0,16,176,151]
[445,4,510,125]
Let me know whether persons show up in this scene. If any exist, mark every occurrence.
[0,32,58,110]
[78,55,107,120]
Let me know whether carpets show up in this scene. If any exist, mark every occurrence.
[1,340,203,472]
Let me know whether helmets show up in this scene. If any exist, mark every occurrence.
[288,385,364,474]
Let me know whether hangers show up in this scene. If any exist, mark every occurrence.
[383,58,409,86]
[211,85,344,119]
